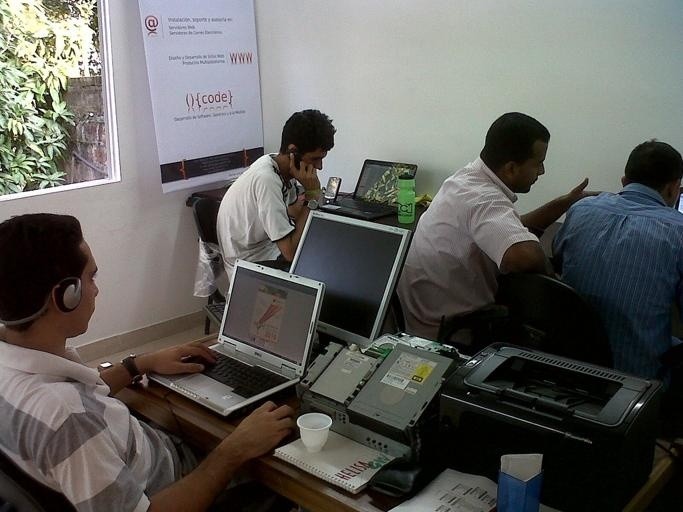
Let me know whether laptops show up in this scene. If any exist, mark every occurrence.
[320,159,418,221]
[146,258,326,417]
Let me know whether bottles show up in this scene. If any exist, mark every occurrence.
[394,170,418,225]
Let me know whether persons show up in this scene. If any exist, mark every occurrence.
[552,140,682,439]
[216,109,337,285]
[396,112,618,342]
[0,214,297,511]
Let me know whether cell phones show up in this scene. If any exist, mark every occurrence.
[324,176,342,200]
[287,147,308,173]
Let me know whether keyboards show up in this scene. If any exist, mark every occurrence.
[202,302,226,330]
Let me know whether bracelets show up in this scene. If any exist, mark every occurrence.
[121,353,143,385]
[304,190,319,195]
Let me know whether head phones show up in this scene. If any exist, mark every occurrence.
[0,277,82,326]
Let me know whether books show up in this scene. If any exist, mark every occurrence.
[272,430,395,495]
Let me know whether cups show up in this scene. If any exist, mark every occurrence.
[296,413,333,455]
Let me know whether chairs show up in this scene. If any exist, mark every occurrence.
[0,465,50,511]
[191,195,226,335]
[506,271,584,358]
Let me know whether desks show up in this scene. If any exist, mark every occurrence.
[184,186,564,263]
[108,331,682,511]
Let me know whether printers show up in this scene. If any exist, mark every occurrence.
[439,342,664,512]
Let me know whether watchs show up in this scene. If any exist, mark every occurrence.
[303,199,319,210]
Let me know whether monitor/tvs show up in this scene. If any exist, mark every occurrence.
[675,187,683,213]
[288,210,413,355]
